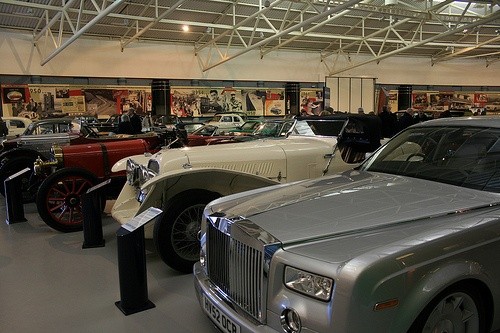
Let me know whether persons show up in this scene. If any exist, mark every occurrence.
[118,108,142,135]
[301,105,486,138]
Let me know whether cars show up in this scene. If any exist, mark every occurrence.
[0,114,296,233]
[109,114,459,274]
[0,116,294,165]
[194,114,500,332]
